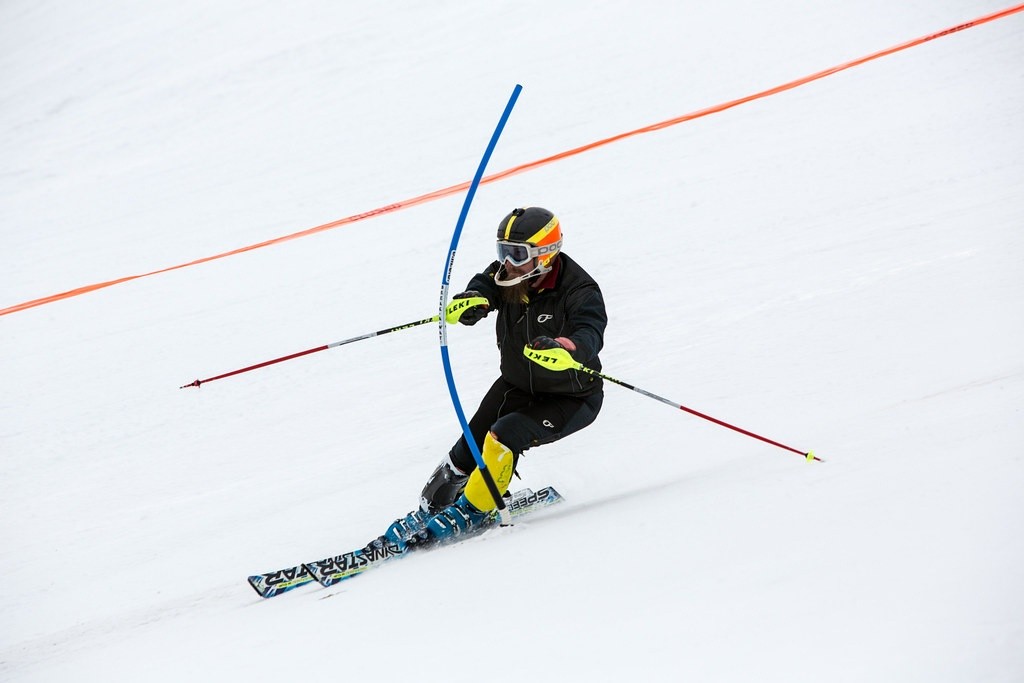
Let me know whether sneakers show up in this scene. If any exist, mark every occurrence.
[383,505,440,544]
[424,492,494,540]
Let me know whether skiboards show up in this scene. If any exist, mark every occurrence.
[243,483,567,604]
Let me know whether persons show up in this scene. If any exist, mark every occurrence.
[388,209,607,550]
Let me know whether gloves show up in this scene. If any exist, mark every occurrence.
[452,290,489,326]
[528,335,577,358]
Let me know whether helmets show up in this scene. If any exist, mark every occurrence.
[496,206,564,272]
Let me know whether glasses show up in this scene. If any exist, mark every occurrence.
[495,240,540,267]
